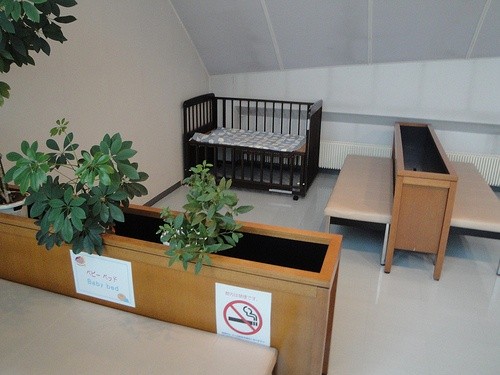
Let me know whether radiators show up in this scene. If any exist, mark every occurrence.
[318,140,500,189]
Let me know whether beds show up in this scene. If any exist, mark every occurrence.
[179,93,324,198]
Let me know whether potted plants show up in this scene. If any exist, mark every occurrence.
[2,125,148,255]
[0,1,77,217]
[154,161,252,273]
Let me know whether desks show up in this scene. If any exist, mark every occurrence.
[0,278,277,375]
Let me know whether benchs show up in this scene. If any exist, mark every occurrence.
[326,124,499,274]
